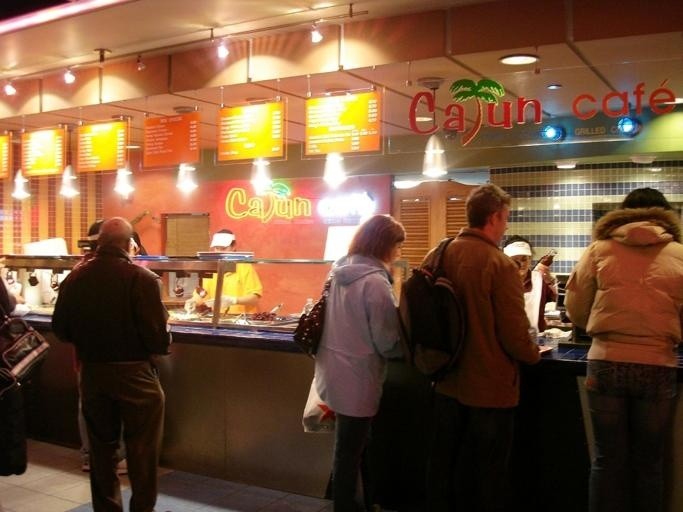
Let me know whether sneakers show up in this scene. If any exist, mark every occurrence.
[117,460,127,474]
[81,463,90,470]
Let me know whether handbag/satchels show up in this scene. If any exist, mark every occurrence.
[1,315,49,399]
[292,295,325,360]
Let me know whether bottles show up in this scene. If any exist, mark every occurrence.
[532,248,558,271]
[303,297,315,318]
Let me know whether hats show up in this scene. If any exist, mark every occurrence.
[503,241,532,257]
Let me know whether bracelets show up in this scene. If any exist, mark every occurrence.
[546,277,560,288]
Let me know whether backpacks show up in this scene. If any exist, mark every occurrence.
[402,270,466,379]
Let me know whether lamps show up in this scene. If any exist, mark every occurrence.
[616,117,641,137]
[1,268,217,300]
[111,114,134,198]
[173,106,198,197]
[323,88,347,188]
[419,78,446,178]
[59,123,80,199]
[0,1,369,95]
[539,124,567,142]
[245,96,271,195]
[11,128,30,201]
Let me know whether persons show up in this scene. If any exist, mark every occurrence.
[562,187,683,512]
[0,279,28,478]
[183,229,264,315]
[419,183,541,512]
[49,216,171,512]
[313,213,408,512]
[501,234,561,337]
